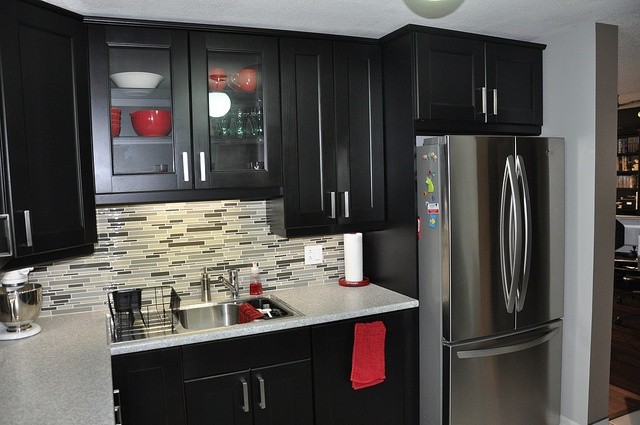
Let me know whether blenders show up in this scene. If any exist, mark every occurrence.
[0,266,47,341]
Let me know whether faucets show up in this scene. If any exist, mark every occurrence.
[217,270,239,299]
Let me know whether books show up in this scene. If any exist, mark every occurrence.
[616,176,637,189]
[617,137,640,154]
[616,156,638,172]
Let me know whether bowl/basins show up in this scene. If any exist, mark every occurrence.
[110,108,121,137]
[109,71,164,97]
[129,109,172,137]
[154,164,168,173]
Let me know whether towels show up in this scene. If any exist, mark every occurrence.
[350,322,386,391]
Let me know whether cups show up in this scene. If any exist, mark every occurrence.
[231,68,257,92]
[217,107,263,139]
[208,68,227,91]
[227,77,241,92]
[250,162,263,170]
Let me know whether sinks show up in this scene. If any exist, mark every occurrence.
[108,313,179,343]
[173,303,253,331]
[246,297,291,320]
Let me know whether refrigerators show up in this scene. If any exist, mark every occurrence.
[415,134,566,425]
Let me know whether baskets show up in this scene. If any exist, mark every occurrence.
[106,286,181,341]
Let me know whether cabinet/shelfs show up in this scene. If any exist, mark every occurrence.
[383,23,543,228]
[83,23,284,205]
[1,0,99,272]
[181,327,314,424]
[110,348,187,425]
[281,33,386,236]
[616,108,640,214]
[311,308,420,425]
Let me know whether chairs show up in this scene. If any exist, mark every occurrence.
[613,218,640,324]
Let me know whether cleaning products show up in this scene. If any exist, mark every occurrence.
[249,261,263,295]
